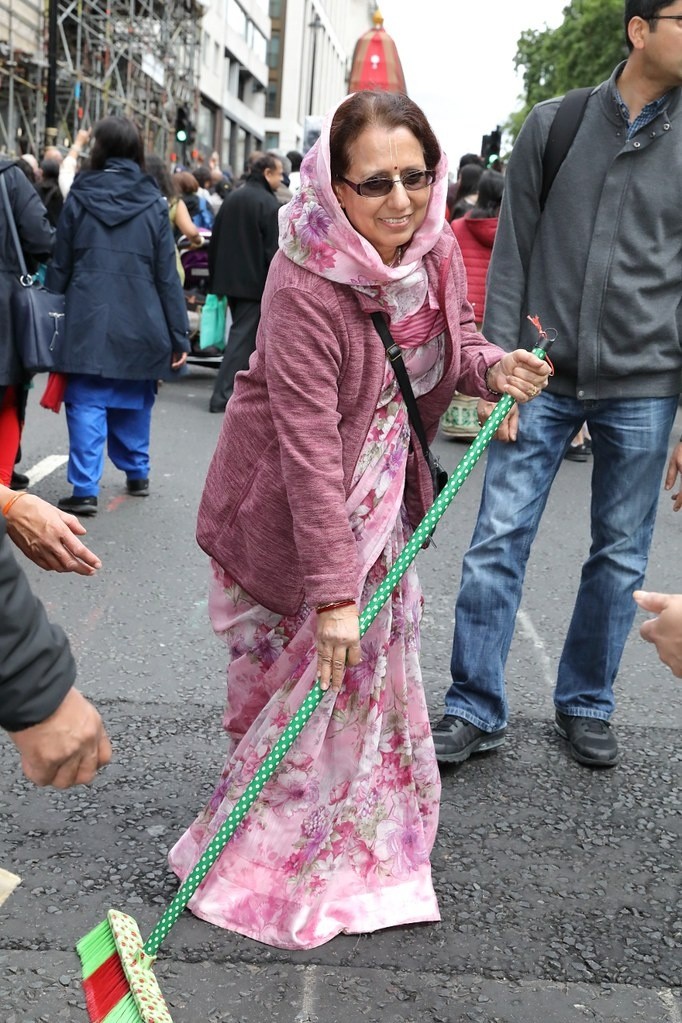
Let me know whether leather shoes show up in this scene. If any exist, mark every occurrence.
[565,438,593,461]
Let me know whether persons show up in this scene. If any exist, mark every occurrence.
[144,150,305,414]
[45,114,191,518]
[633,591,682,678]
[169,91,551,950]
[430,0,682,765]
[440,153,592,462]
[0,126,112,790]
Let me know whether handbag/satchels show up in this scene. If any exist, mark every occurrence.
[419,451,448,550]
[199,294,227,350]
[12,285,66,373]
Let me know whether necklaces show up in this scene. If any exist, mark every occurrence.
[388,247,401,268]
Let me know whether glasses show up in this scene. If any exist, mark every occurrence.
[339,170,435,198]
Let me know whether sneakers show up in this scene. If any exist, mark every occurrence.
[126,478,149,496]
[553,709,618,765]
[58,496,97,513]
[432,715,505,763]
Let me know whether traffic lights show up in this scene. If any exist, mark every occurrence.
[485,132,499,170]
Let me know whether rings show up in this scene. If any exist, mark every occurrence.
[478,422,481,425]
[529,386,537,397]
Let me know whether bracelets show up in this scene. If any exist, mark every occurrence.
[2,492,27,520]
[484,360,501,395]
[193,234,205,247]
[315,599,356,615]
[71,144,82,152]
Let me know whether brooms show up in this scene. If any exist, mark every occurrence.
[74,326,559,1023]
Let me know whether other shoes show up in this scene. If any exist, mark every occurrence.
[210,406,225,412]
[11,472,29,489]
